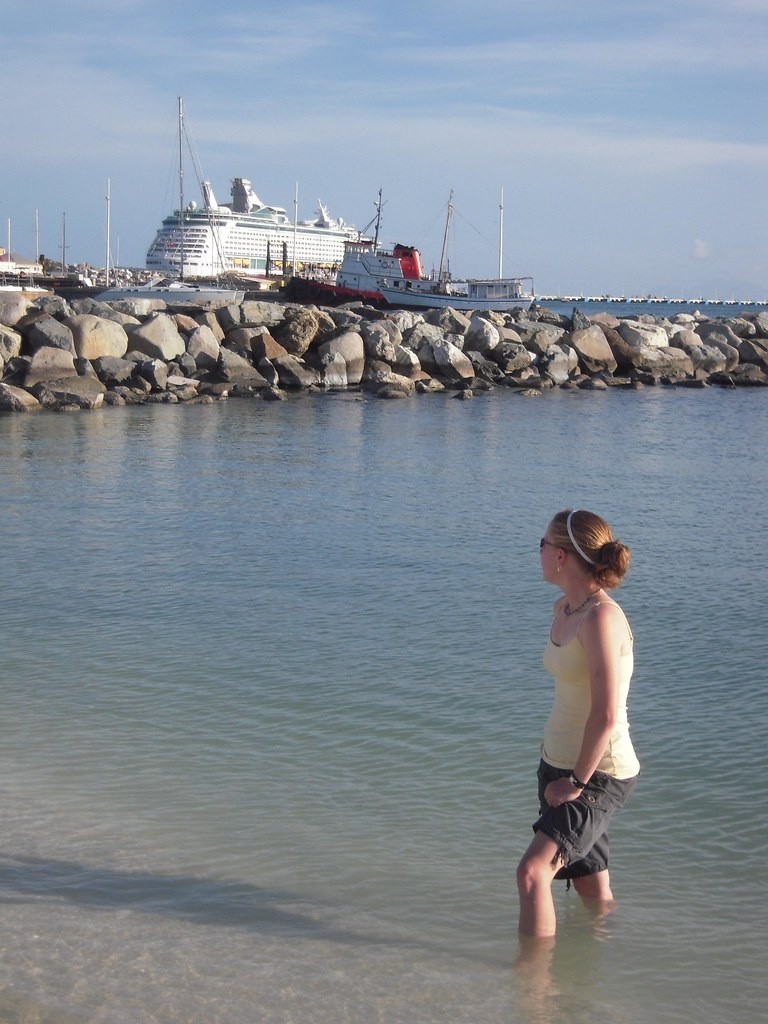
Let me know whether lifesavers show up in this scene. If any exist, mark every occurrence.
[382,262,388,268]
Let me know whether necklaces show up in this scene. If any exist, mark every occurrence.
[563,589,601,616]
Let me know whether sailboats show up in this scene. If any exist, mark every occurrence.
[0,95,247,307]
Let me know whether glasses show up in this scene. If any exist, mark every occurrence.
[539,538,568,553]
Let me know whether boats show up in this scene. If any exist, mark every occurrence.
[144,176,378,278]
[284,275,383,307]
[336,189,534,315]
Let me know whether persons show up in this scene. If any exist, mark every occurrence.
[516,510,641,939]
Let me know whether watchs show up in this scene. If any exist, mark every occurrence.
[569,772,586,789]
